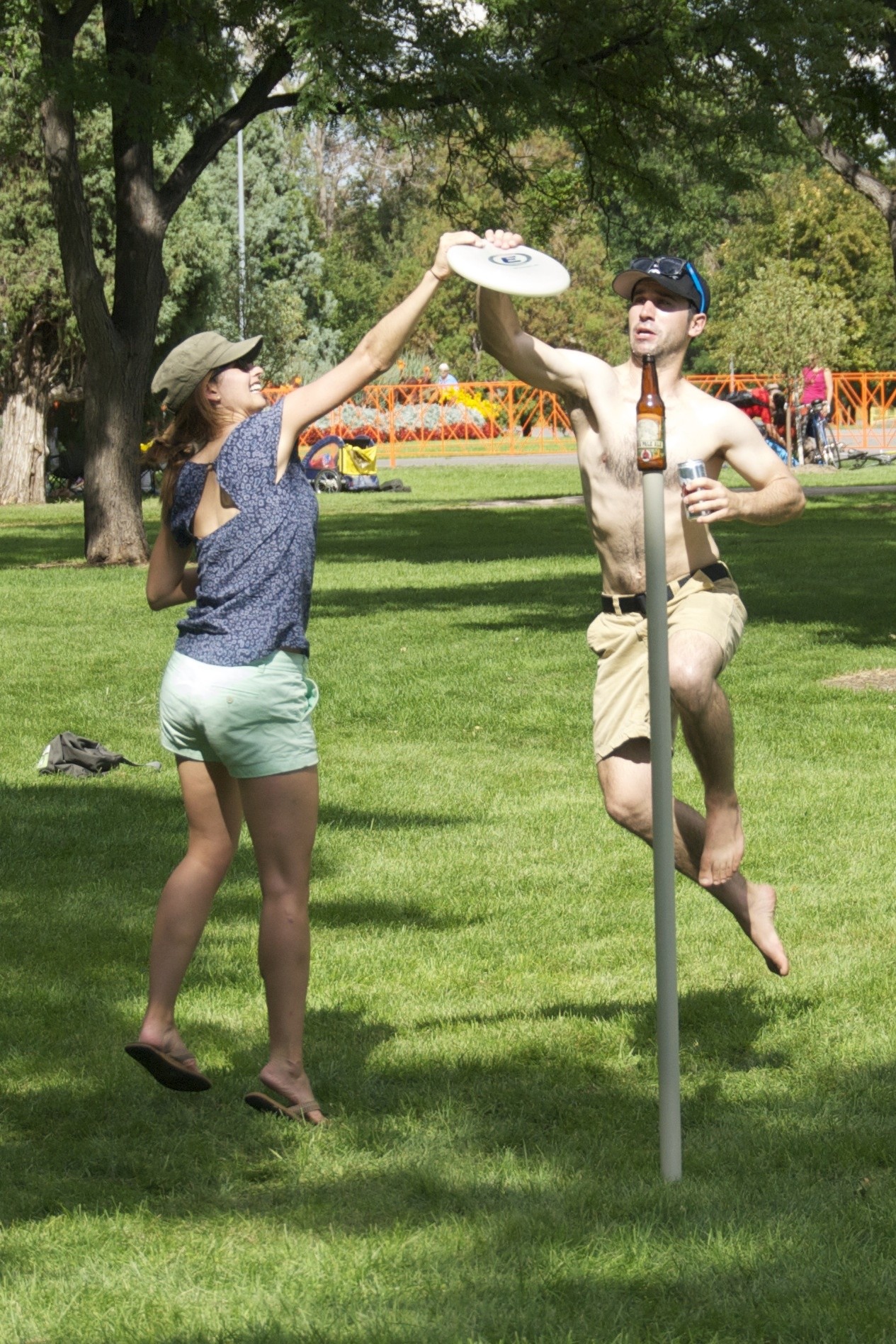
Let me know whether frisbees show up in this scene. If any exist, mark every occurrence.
[446,239,570,296]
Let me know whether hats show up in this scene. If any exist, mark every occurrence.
[144,330,268,415]
[612,253,711,314]
[439,362,449,371]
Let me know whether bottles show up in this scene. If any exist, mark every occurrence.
[636,353,668,471]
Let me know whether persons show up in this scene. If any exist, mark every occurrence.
[125,230,484,1125]
[476,230,807,977]
[435,361,462,407]
[721,351,840,472]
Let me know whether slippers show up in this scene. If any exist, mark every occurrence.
[123,1042,212,1096]
[242,1092,330,1127]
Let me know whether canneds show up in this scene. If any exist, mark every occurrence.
[677,459,714,523]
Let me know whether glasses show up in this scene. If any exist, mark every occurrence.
[629,256,706,313]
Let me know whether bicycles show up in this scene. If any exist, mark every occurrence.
[796,398,842,469]
[832,445,896,471]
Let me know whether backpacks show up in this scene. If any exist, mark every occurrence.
[725,389,758,408]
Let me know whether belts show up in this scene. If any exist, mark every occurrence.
[600,563,732,613]
